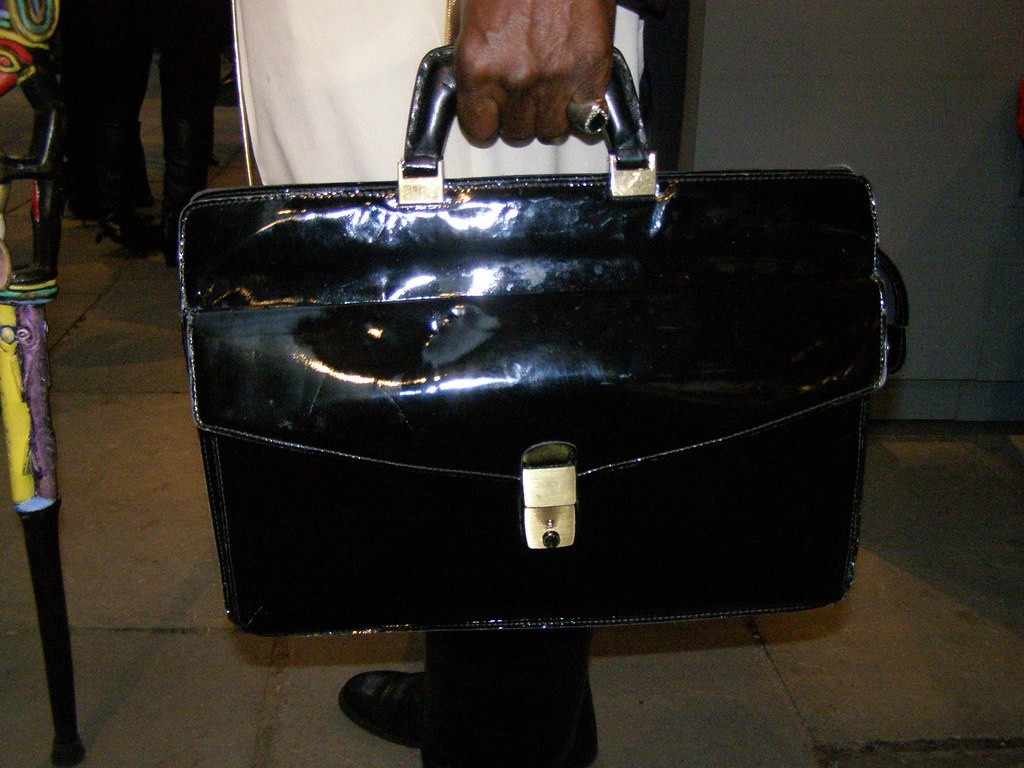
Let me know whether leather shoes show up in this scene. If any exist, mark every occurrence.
[339,671,425,749]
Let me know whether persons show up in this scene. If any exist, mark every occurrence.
[231,0,646,768]
[60,0,213,267]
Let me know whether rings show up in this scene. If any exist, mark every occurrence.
[584,105,609,135]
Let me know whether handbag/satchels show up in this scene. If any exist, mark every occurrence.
[177,42,888,636]
